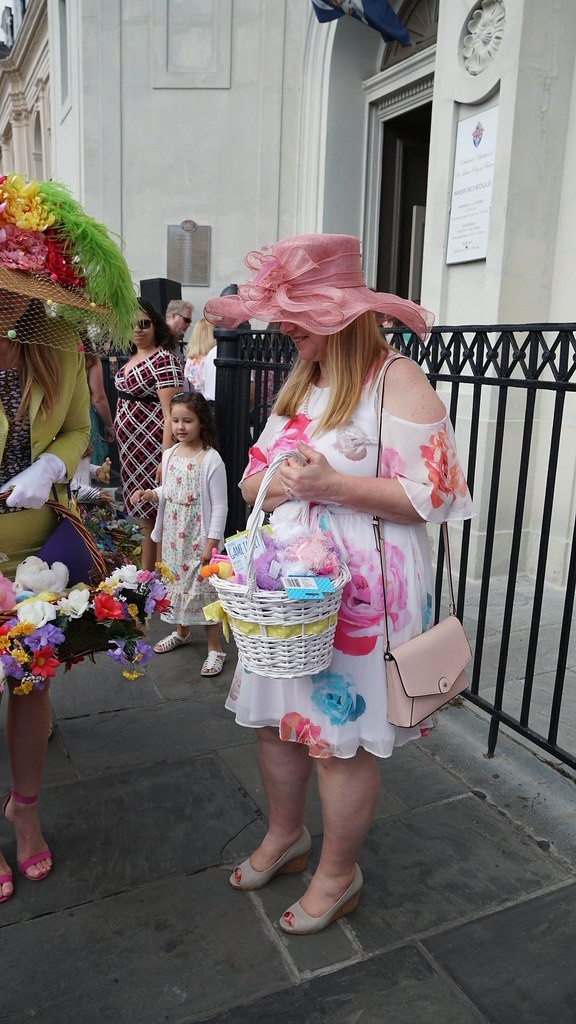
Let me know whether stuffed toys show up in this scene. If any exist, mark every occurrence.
[98,457,112,484]
[200,530,341,590]
[12,554,70,594]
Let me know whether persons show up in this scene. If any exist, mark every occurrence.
[368,287,420,329]
[0,176,141,901]
[202,235,475,937]
[55,307,114,526]
[112,297,183,578]
[130,393,229,676]
[70,442,112,503]
[166,299,193,338]
[183,319,299,443]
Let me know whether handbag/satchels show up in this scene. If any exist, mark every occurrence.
[384,617,472,726]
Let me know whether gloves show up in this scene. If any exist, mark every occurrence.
[0,453,66,510]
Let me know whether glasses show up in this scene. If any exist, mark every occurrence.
[173,312,193,323]
[130,319,153,331]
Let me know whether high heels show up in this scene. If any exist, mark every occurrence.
[230,827,312,890]
[278,863,363,935]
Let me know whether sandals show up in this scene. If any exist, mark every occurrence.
[153,629,193,654]
[202,650,226,676]
[0,874,15,903]
[2,791,54,880]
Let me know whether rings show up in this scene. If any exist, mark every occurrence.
[288,488,290,494]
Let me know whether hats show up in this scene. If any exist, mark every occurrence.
[0,176,138,356]
[202,234,436,342]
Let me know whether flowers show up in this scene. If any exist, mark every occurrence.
[0,171,147,356]
[80,504,148,570]
[0,563,175,697]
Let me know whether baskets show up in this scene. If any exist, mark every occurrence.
[208,451,352,679]
[0,491,143,664]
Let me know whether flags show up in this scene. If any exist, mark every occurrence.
[311,0,411,47]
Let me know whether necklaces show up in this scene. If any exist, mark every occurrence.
[304,372,321,420]
